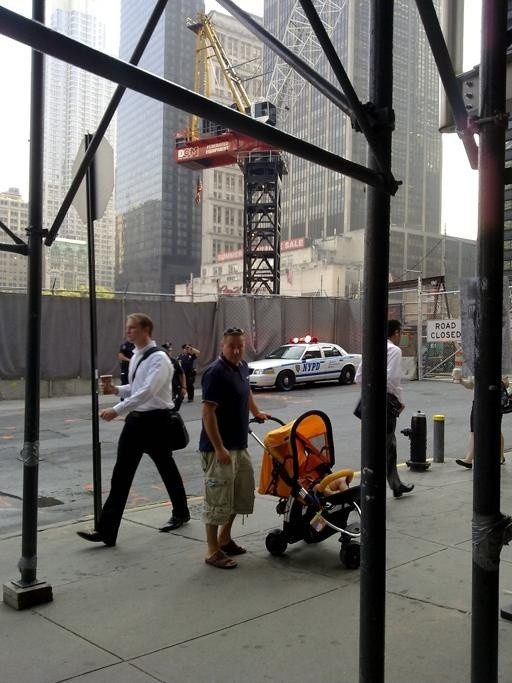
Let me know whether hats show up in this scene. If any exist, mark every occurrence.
[161,343,172,349]
[182,343,190,350]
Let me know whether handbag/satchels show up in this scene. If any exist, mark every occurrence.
[502,393,512,414]
[354,393,401,433]
[168,413,189,451]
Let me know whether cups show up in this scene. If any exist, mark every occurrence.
[99,374,113,396]
[452,368,461,384]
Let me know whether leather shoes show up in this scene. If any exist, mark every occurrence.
[77,531,116,546]
[394,484,414,498]
[159,517,190,532]
[456,460,472,469]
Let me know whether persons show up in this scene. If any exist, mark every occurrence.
[451,376,510,469]
[76,312,191,546]
[353,320,415,497]
[311,472,349,498]
[176,343,201,402]
[161,343,187,412]
[198,327,272,569]
[118,340,136,402]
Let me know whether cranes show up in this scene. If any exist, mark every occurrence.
[172,1,353,295]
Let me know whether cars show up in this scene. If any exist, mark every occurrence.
[248,334,363,392]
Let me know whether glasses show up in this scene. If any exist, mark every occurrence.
[224,328,243,334]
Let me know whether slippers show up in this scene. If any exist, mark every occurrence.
[221,542,246,554]
[204,551,237,568]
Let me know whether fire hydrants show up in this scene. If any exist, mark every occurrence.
[399,410,431,471]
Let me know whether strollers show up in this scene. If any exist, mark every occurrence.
[248,410,361,568]
